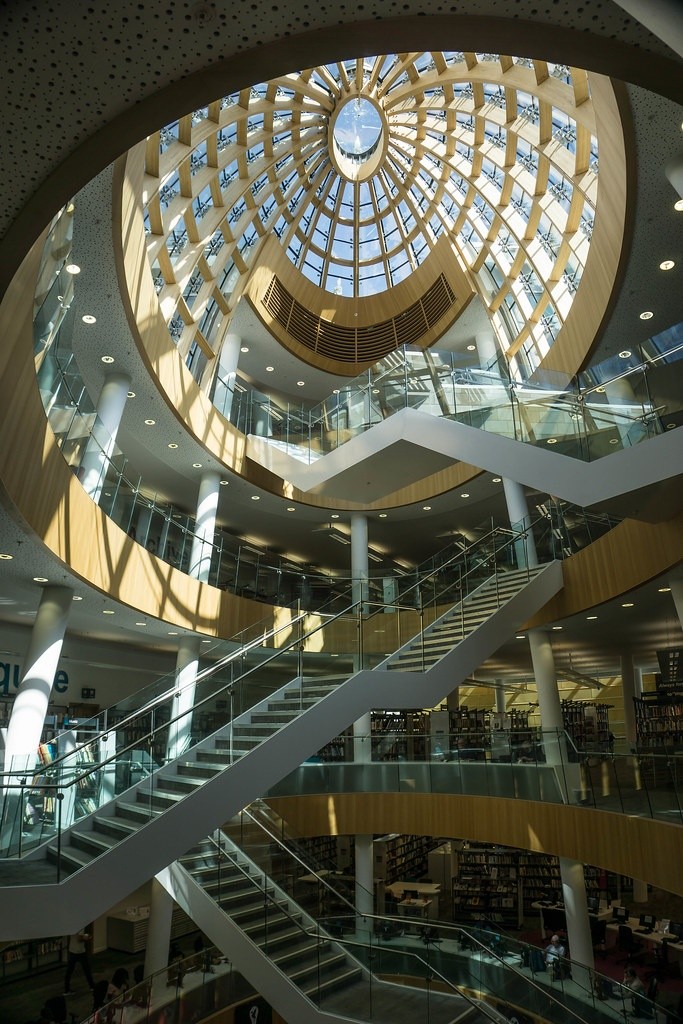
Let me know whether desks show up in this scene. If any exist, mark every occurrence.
[531,899,614,939]
[606,922,683,954]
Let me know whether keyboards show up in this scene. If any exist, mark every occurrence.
[604,991,621,999]
[607,920,622,924]
[661,938,678,943]
[588,909,598,915]
[539,901,549,907]
[634,929,651,934]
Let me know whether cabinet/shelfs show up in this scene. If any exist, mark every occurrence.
[451,849,564,931]
[583,865,634,906]
[335,836,355,869]
[283,836,338,876]
[373,834,445,915]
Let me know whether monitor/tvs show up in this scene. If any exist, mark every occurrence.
[523,949,548,971]
[635,994,653,1019]
[600,977,613,998]
[457,922,477,950]
[612,907,630,923]
[423,922,439,942]
[668,920,683,941]
[552,890,558,905]
[587,895,600,912]
[490,937,507,956]
[639,913,656,932]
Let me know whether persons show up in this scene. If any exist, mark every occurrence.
[612,965,643,998]
[542,935,567,973]
